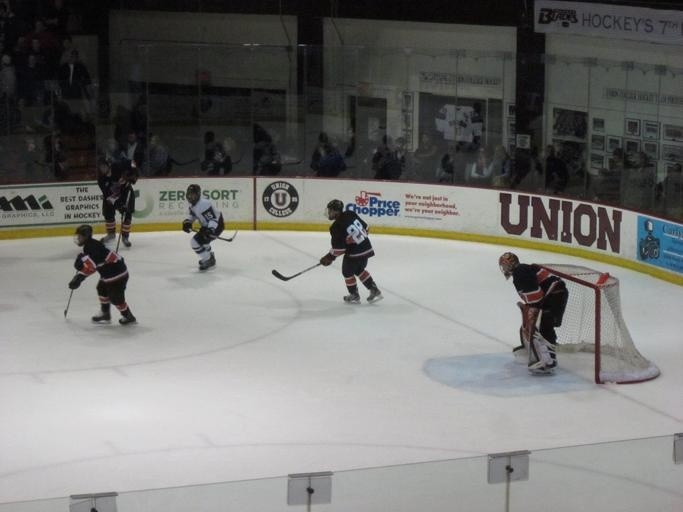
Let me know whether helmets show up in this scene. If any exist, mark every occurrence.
[498,252,520,280]
[96,156,112,175]
[185,184,200,205]
[327,199,343,220]
[73,225,92,246]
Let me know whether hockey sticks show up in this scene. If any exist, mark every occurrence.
[272,263,321,281]
[63,270,79,317]
[192,230,237,242]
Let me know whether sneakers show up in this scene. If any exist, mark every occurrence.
[343,293,359,302]
[92,314,110,321]
[366,286,380,301]
[121,237,131,247]
[528,358,555,370]
[100,234,116,243]
[119,316,136,324]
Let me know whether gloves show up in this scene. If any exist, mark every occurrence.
[205,228,216,241]
[320,253,336,266]
[182,219,193,233]
[74,254,83,271]
[68,274,86,289]
[112,199,127,214]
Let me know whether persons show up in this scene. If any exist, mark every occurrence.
[248,127,284,176]
[395,128,683,224]
[1,0,149,185]
[321,199,382,303]
[310,132,347,178]
[199,131,234,178]
[96,130,138,247]
[138,133,175,177]
[498,252,569,374]
[181,185,225,269]
[370,134,404,181]
[67,225,137,326]
